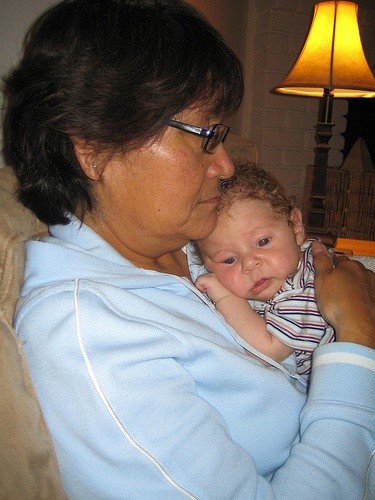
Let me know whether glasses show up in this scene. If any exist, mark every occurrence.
[167,119,230,154]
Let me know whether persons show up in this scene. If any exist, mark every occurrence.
[0,0,375,500]
[193,159,336,387]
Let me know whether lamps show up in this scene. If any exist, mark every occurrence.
[270,0,375,249]
